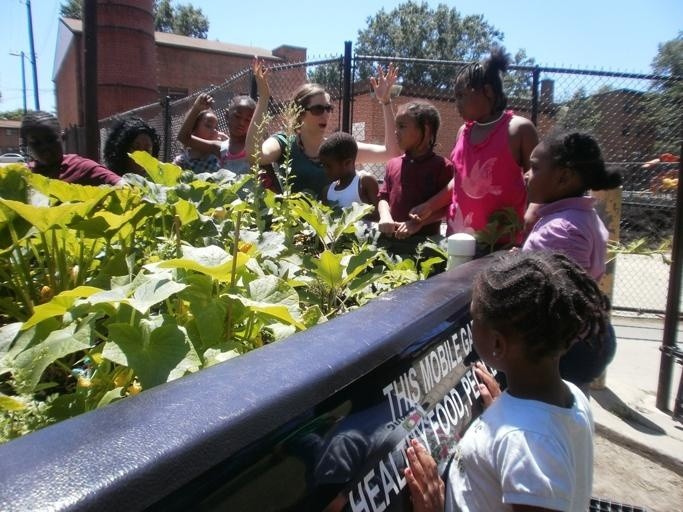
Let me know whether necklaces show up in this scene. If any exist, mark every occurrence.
[403,152,436,164]
[473,109,505,126]
[308,155,320,160]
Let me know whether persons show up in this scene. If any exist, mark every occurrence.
[304,397,461,512]
[522,132,623,285]
[409,47,539,244]
[404,250,616,512]
[318,132,379,221]
[172,110,229,175]
[245,56,399,199]
[177,93,273,190]
[104,117,162,176]
[377,100,453,241]
[21,112,122,186]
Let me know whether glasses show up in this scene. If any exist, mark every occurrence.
[305,103,334,117]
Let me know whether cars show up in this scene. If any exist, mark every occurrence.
[0,153,26,165]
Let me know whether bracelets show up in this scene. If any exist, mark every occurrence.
[380,98,392,105]
